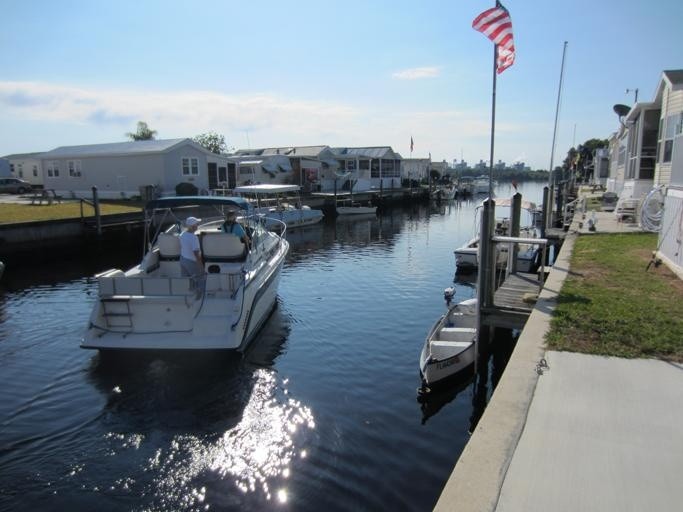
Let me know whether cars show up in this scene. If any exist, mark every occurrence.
[0,177,32,194]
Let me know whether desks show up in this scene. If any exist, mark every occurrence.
[590,182,605,194]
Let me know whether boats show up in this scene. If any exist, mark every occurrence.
[419,287,477,385]
[455,175,497,196]
[336,199,378,214]
[454,198,541,272]
[429,185,456,199]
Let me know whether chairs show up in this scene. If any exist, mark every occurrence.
[154,231,246,261]
[617,197,639,223]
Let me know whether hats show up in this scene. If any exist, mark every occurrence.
[186,216,200,227]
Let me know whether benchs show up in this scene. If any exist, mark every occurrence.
[31,188,62,207]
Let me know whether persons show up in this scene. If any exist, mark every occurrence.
[178,217,204,296]
[219,210,249,244]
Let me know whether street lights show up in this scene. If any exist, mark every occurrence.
[623,88,638,104]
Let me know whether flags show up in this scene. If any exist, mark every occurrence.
[472,0,514,74]
